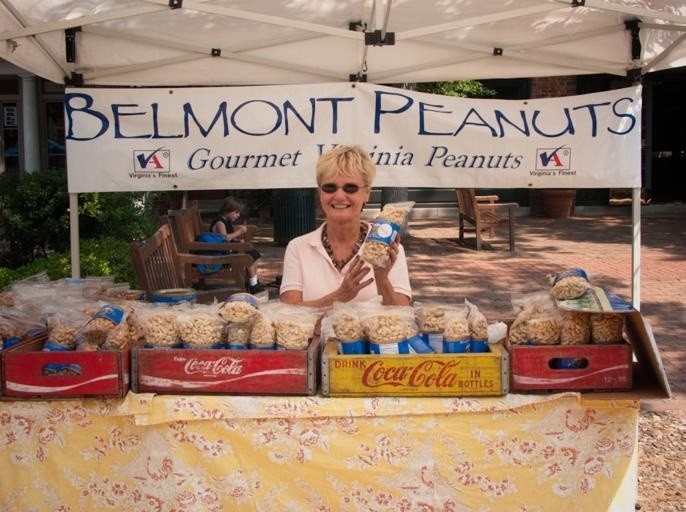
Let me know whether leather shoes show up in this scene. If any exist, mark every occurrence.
[249,283,265,294]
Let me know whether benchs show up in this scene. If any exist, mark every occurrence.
[126,200,257,305]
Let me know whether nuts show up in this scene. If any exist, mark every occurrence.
[1,205,623,349]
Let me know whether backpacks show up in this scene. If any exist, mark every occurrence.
[197,232,228,274]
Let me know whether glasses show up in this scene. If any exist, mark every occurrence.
[319,184,367,193]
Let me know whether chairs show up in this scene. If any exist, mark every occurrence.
[455,187,520,251]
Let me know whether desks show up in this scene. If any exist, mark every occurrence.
[2,394,641,512]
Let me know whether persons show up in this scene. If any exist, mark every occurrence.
[279,144,412,307]
[210,197,264,294]
